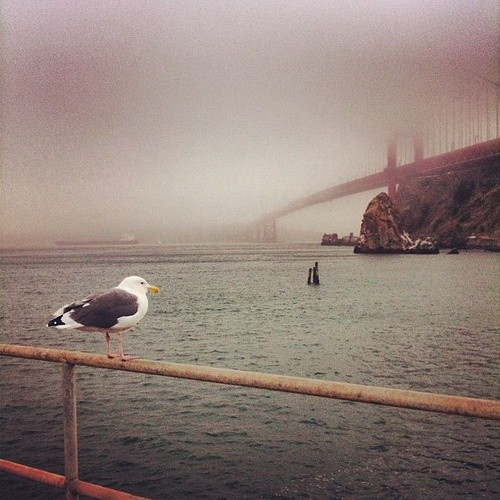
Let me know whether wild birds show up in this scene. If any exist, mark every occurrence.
[46,276,159,363]
